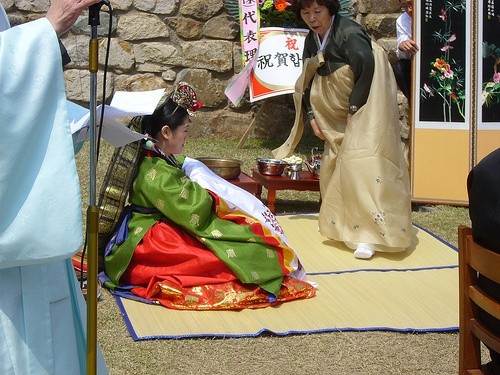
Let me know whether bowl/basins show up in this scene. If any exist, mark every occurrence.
[286,164,302,180]
[256,158,287,176]
[195,156,244,180]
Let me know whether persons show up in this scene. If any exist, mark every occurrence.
[394,0,419,96]
[467,148,500,366]
[99,83,318,310]
[0,0,108,375]
[272,0,412,259]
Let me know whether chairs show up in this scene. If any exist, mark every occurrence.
[457,225,500,375]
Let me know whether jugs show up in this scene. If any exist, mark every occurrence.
[305,147,324,179]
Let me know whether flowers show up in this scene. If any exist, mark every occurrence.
[260,0,302,29]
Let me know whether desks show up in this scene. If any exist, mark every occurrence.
[251,168,319,215]
[228,170,262,201]
